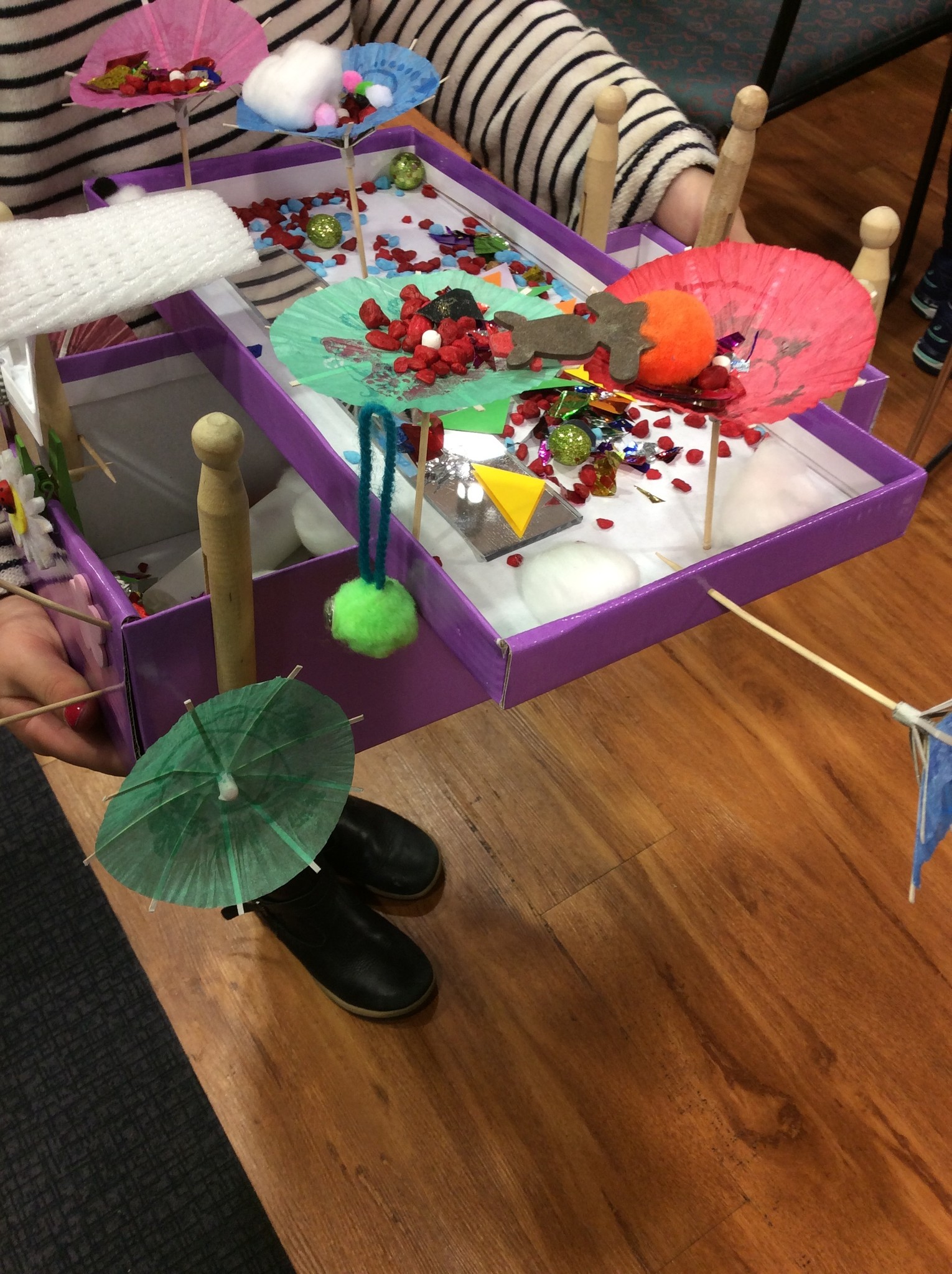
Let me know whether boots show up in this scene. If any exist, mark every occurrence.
[314,795,441,899]
[250,867,437,1020]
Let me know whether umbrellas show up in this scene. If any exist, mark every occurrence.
[56,3,881,456]
[87,658,354,913]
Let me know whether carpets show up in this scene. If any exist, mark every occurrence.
[0,721,300,1274]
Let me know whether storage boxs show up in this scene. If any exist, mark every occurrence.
[0,122,931,786]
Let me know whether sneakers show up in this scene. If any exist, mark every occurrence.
[912,294,951,377]
[911,247,948,319]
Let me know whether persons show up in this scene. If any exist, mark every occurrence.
[0,0,763,1025]
[904,157,952,372]
